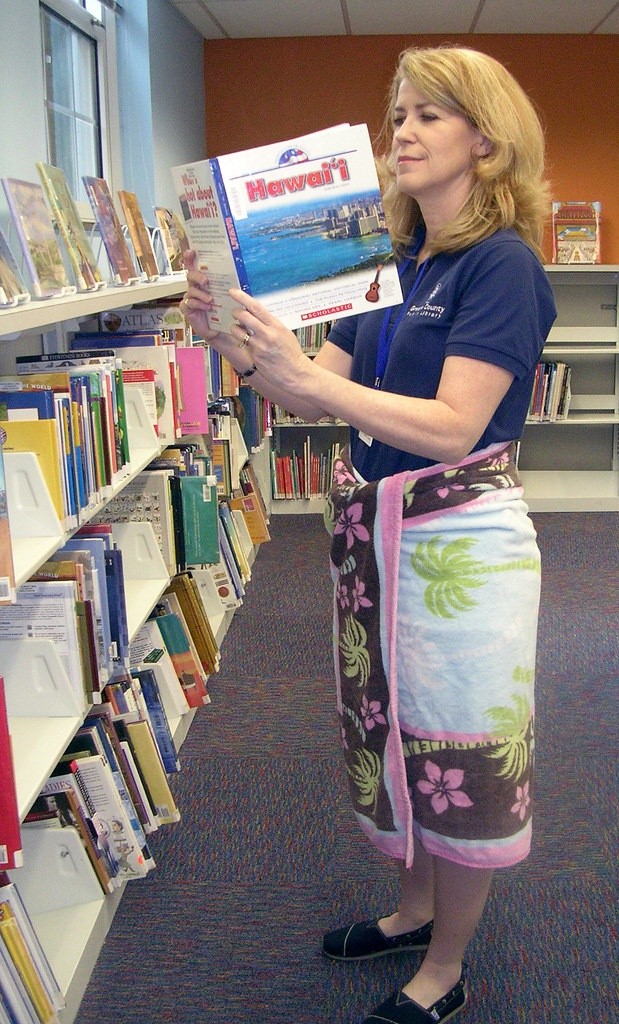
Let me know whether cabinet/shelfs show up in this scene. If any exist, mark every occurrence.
[0,273,269,1024]
[271,263,619,516]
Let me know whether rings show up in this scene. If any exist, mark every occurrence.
[184,298,190,308]
[242,334,250,345]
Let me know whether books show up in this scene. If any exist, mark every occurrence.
[0,294,239,605]
[117,189,159,278]
[0,225,28,309]
[525,369,572,424]
[1,176,69,297]
[81,175,138,285]
[550,201,602,264]
[293,319,338,352]
[514,442,521,468]
[270,434,340,500]
[0,460,274,1023]
[34,160,104,291]
[266,400,344,435]
[1,387,266,719]
[171,122,404,335]
[152,205,190,274]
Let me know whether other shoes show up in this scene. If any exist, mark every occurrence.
[322,911,434,961]
[362,959,469,1024]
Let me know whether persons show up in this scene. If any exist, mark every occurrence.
[179,44,558,1024]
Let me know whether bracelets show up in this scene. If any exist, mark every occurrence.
[235,363,257,379]
